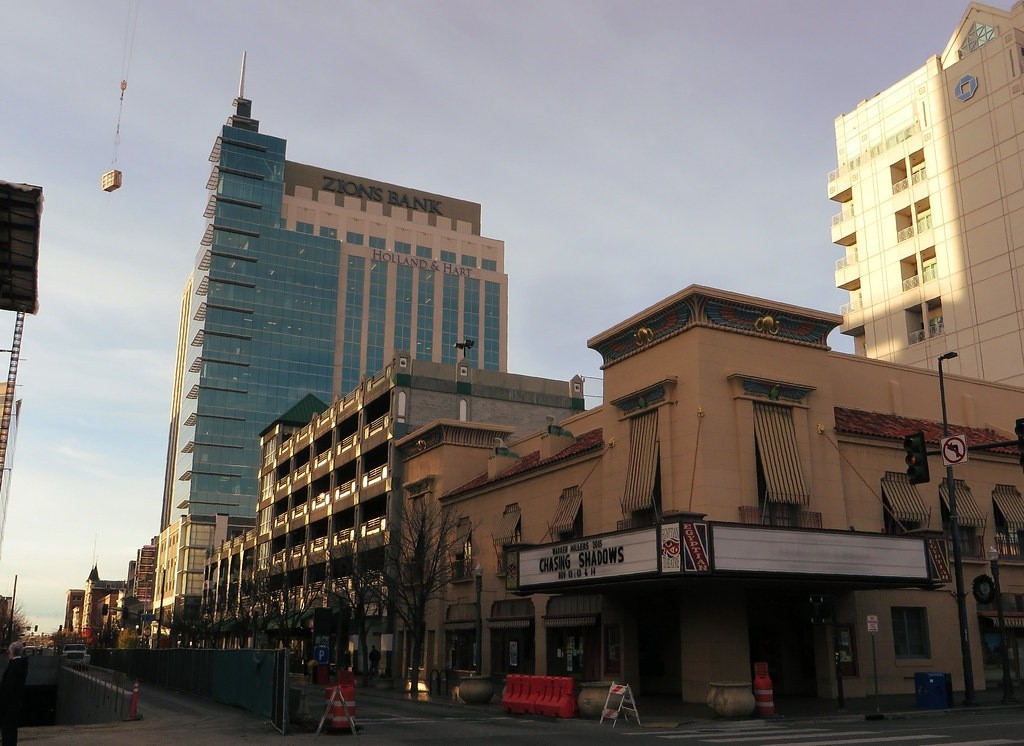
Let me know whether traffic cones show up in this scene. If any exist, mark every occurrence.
[122,678,140,721]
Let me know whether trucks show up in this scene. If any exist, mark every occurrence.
[63,642,86,667]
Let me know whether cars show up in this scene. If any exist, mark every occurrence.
[22,640,36,651]
[69,659,88,672]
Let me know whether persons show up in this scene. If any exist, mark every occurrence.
[369,645,381,679]
[39,646,42,655]
[344,650,351,670]
[0,641,30,746]
[32,648,37,655]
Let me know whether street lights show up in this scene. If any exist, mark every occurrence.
[937,351,977,706]
[986,547,1016,707]
[475,560,484,676]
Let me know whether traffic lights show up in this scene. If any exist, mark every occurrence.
[900,431,930,485]
[135,623,141,635]
[1014,418,1024,469]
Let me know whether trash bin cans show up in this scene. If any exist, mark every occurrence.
[914,671,954,709]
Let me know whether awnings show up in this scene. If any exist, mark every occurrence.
[753,401,810,526]
[548,485,583,539]
[992,485,1024,534]
[881,470,931,532]
[621,411,660,523]
[450,518,472,560]
[939,479,987,544]
[493,504,521,544]
